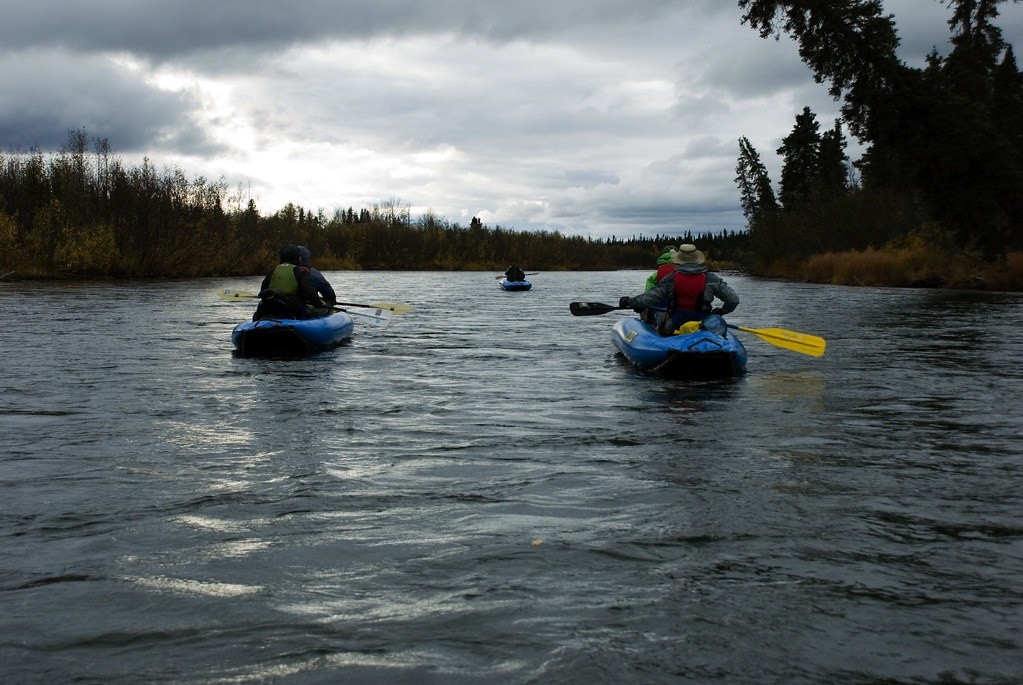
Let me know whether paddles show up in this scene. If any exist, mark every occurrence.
[570,300,621,317]
[650,306,825,358]
[216,287,409,327]
[495,272,539,280]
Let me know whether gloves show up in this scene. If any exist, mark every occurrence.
[619,296,630,308]
[712,307,724,317]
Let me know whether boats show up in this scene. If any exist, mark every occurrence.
[611,314,749,376]
[232,307,353,353]
[497,279,532,290]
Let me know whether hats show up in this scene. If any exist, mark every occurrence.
[279,246,299,263]
[300,246,312,260]
[672,244,706,265]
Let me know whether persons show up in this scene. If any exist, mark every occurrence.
[619,242,739,338]
[506,261,526,282]
[259,245,337,317]
